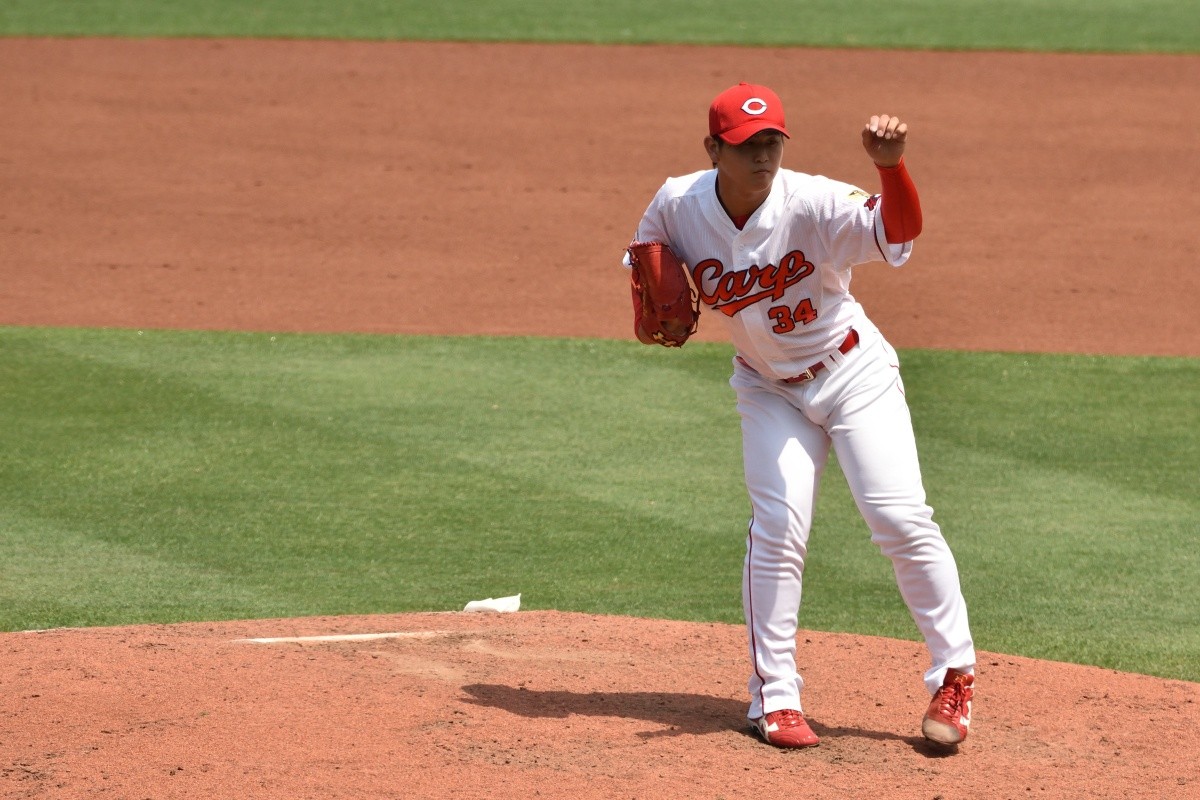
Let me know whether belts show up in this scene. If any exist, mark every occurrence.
[736,329,861,384]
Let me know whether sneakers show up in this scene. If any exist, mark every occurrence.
[750,709,820,747]
[922,668,977,746]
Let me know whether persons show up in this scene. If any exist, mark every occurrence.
[618,81,977,744]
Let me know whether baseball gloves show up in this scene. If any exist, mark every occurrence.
[620,239,702,350]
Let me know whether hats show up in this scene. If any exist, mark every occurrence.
[709,82,790,146]
